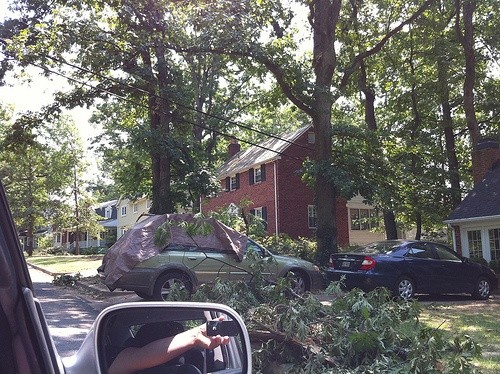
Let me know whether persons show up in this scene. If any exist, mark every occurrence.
[103,316,230,374]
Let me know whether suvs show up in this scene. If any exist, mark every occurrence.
[104,214,323,306]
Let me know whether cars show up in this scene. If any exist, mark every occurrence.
[328,237,497,304]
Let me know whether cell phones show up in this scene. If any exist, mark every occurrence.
[207,321,238,336]
